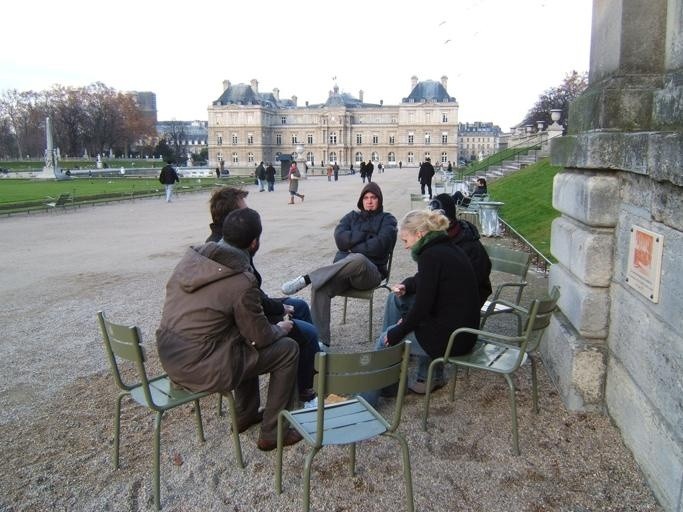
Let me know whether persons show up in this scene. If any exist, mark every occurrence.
[417,157,487,207]
[408,191,494,398]
[355,208,480,412]
[398,160,402,170]
[281,183,399,352]
[254,152,385,193]
[215,166,220,178]
[285,161,306,205]
[64,169,72,176]
[204,186,322,402]
[159,161,180,203]
[155,206,304,452]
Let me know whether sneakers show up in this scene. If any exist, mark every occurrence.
[318,341,328,352]
[258,429,302,451]
[231,406,265,434]
[298,388,317,402]
[409,378,445,394]
[281,275,307,295]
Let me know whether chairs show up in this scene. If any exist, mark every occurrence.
[408,192,489,228]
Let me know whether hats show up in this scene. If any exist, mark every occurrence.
[430,193,456,219]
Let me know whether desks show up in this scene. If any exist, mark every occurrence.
[473,201,504,237]
